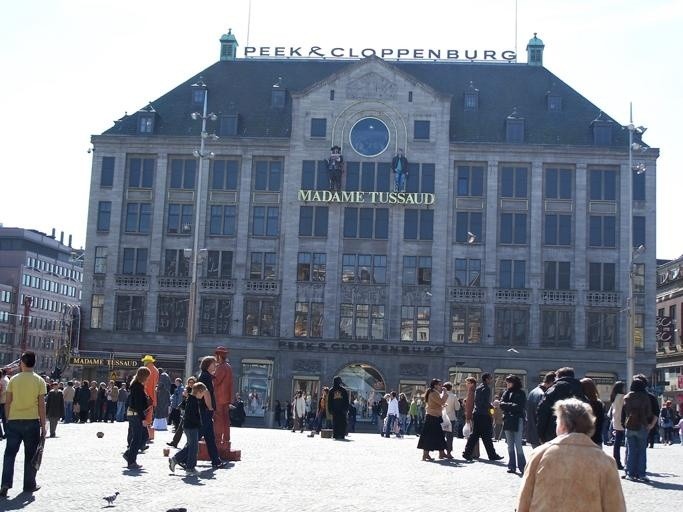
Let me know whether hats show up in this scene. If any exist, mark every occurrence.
[141,355,156,363]
[215,346,229,354]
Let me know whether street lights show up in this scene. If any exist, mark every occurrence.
[620,101,655,395]
[181,108,222,380]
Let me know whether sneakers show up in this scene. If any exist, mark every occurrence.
[637,476,649,482]
[169,458,175,472]
[625,475,634,481]
[380,431,409,438]
[507,469,515,473]
[212,461,228,469]
[186,469,201,477]
[122,450,129,461]
[463,453,473,461]
[488,455,504,461]
[60,417,127,424]
[128,463,142,469]
[277,423,320,437]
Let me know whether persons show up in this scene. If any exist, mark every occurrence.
[270,374,374,443]
[0,351,47,497]
[211,345,232,446]
[229,387,258,427]
[139,355,159,433]
[168,355,232,470]
[391,148,409,193]
[122,365,148,468]
[179,380,205,476]
[324,145,346,192]
[0,368,197,451]
[514,393,627,512]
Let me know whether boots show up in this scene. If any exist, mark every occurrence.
[423,451,434,461]
[439,450,448,459]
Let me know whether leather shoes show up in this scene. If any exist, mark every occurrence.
[23,485,41,492]
[0,488,7,496]
[166,441,178,448]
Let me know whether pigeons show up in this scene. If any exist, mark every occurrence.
[102,492,121,506]
